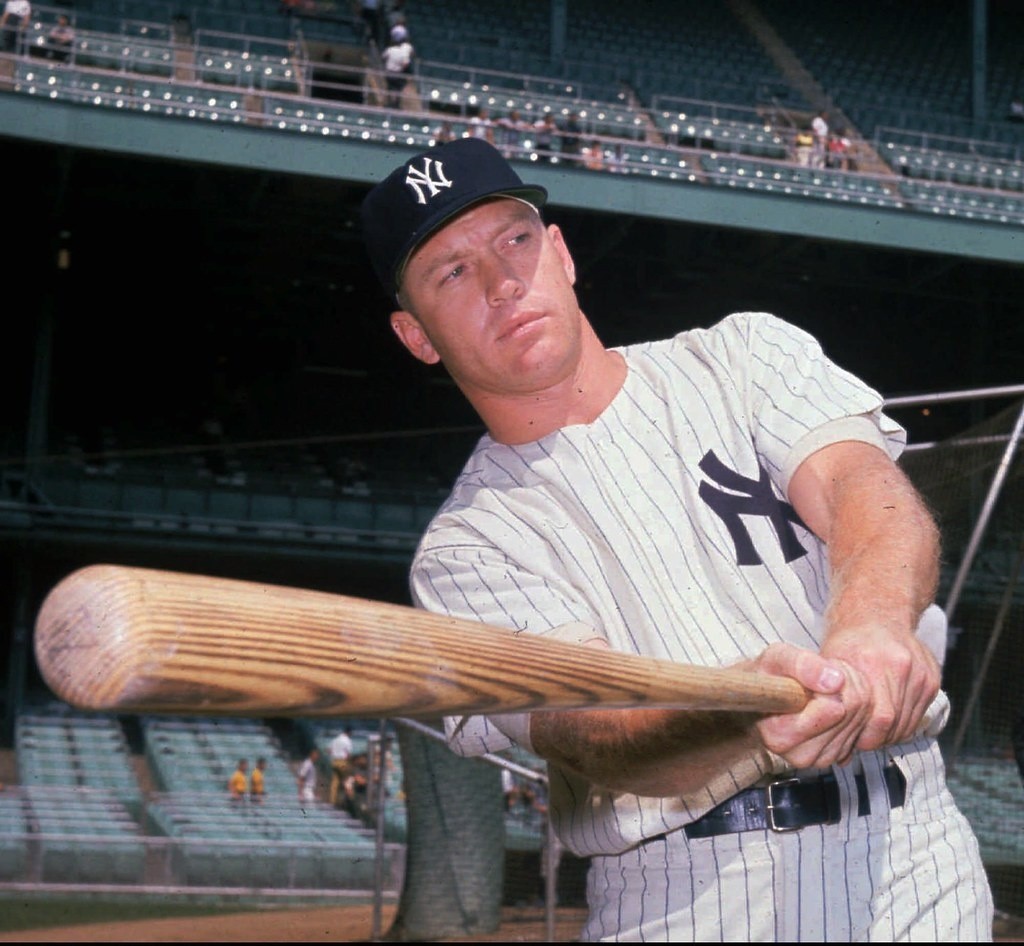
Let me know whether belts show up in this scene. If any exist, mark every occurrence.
[646,763,907,843]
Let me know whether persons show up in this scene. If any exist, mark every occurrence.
[251,758,270,806]
[297,749,321,804]
[227,759,249,808]
[357,0,415,92]
[433,121,456,147]
[0,0,31,54]
[322,725,407,818]
[584,141,628,173]
[33,15,75,60]
[467,107,584,169]
[795,110,858,172]
[500,752,552,832]
[364,133,998,942]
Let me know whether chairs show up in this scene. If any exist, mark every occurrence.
[0,699,1024,892]
[0,0,1024,227]
[31,461,451,544]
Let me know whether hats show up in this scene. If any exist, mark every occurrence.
[357,136,548,301]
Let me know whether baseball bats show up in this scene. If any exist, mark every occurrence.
[36,563,952,740]
